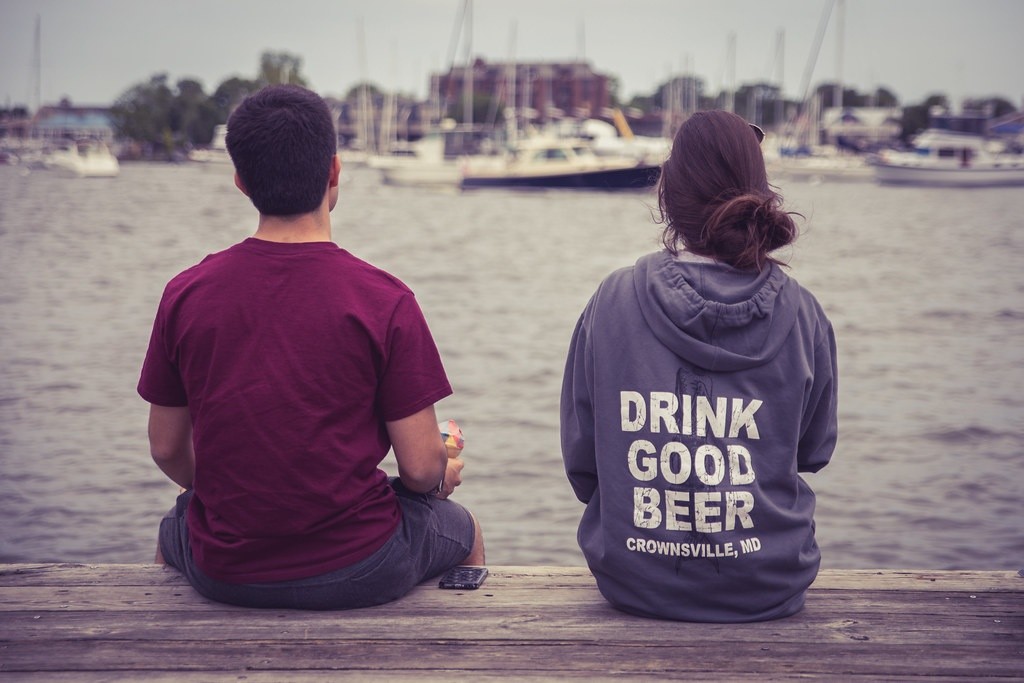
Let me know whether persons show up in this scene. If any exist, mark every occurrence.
[138,82,485,610]
[559,109,837,621]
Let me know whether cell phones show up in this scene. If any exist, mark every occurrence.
[440,566,488,589]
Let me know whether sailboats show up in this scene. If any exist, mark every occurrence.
[0,1,1023,186]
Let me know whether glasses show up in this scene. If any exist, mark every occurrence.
[748,122,765,144]
